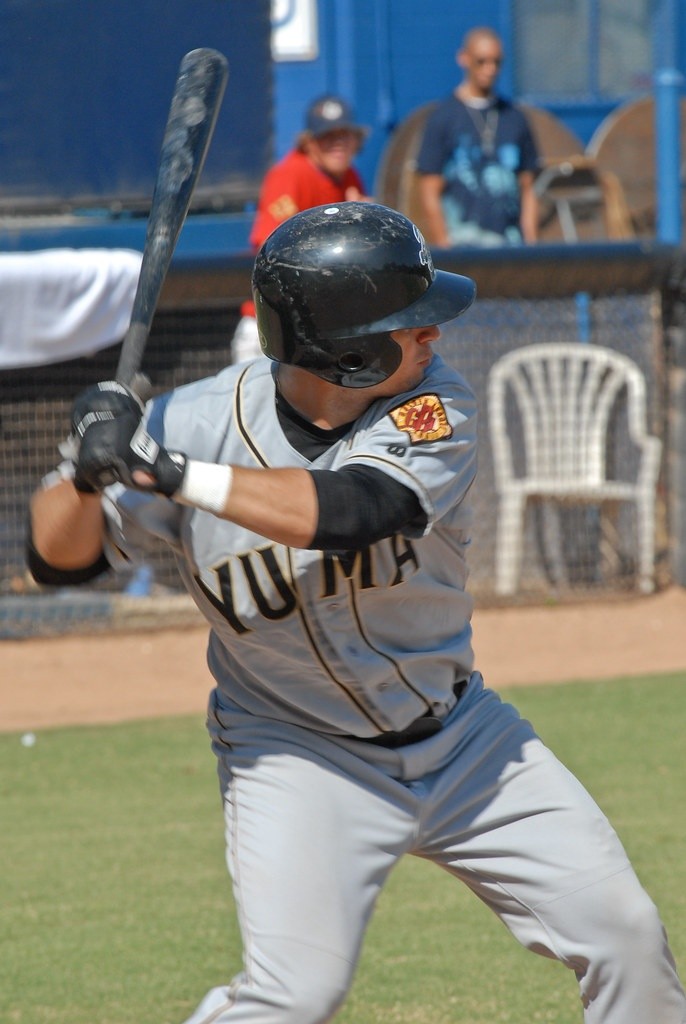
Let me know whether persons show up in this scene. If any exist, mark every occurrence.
[413,27,545,248]
[229,96,375,366]
[24,200,686,1024]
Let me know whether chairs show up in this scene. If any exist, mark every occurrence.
[486,342,663,593]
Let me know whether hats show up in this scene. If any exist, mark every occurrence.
[306,98,364,138]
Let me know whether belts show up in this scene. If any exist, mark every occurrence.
[338,680,467,750]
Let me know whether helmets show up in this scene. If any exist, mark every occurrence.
[253,201,477,389]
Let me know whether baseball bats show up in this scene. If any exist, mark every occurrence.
[96,47,233,486]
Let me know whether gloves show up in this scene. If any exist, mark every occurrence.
[74,416,188,498]
[71,381,145,441]
